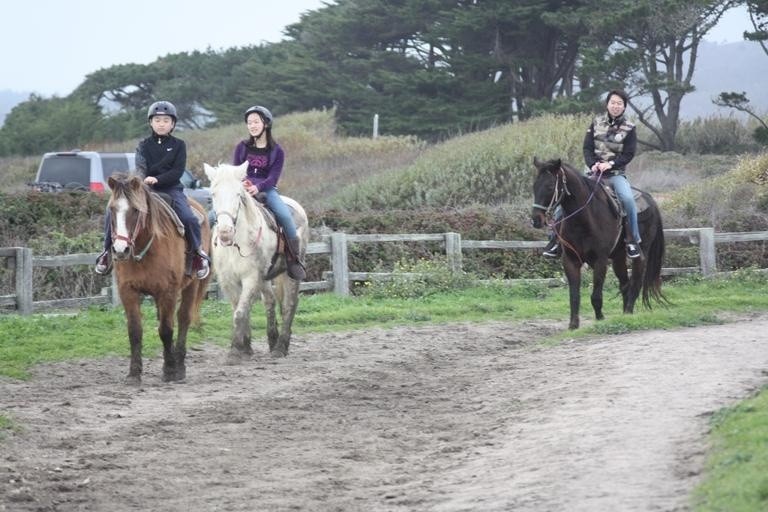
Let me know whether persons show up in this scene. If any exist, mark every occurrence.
[95,101,210,281]
[543,88,642,259]
[207,105,308,280]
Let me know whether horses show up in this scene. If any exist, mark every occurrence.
[107,171,214,393]
[203,159,310,365]
[530,155,674,330]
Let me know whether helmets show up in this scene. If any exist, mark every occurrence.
[147,101,179,120]
[244,105,273,128]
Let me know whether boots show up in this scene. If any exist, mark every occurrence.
[286,237,307,280]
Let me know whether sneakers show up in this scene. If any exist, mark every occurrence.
[194,249,211,278]
[624,242,641,260]
[94,247,113,276]
[543,241,560,258]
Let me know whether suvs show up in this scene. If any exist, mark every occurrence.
[28,147,216,216]
